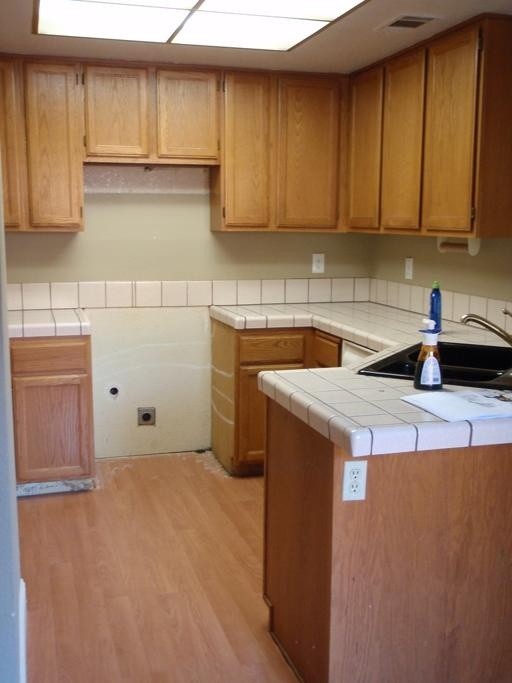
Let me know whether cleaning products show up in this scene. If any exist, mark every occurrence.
[429,281,441,332]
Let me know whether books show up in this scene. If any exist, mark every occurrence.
[401,391,512,423]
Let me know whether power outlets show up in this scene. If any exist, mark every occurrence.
[311,253,326,274]
[138,408,155,426]
[341,459,368,502]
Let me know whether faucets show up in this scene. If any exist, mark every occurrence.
[459,309,512,345]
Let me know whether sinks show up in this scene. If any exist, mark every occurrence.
[393,340,512,369]
[357,356,511,391]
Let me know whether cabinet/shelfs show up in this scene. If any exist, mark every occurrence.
[209,315,313,477]
[346,11,512,240]
[81,56,223,168]
[2,50,87,233]
[9,334,97,486]
[210,66,346,232]
